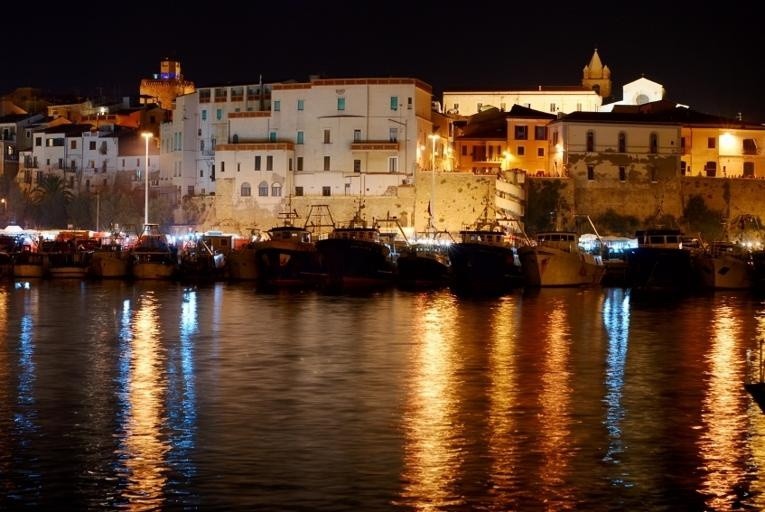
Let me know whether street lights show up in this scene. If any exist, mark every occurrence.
[141,132,154,237]
[427,134,441,228]
